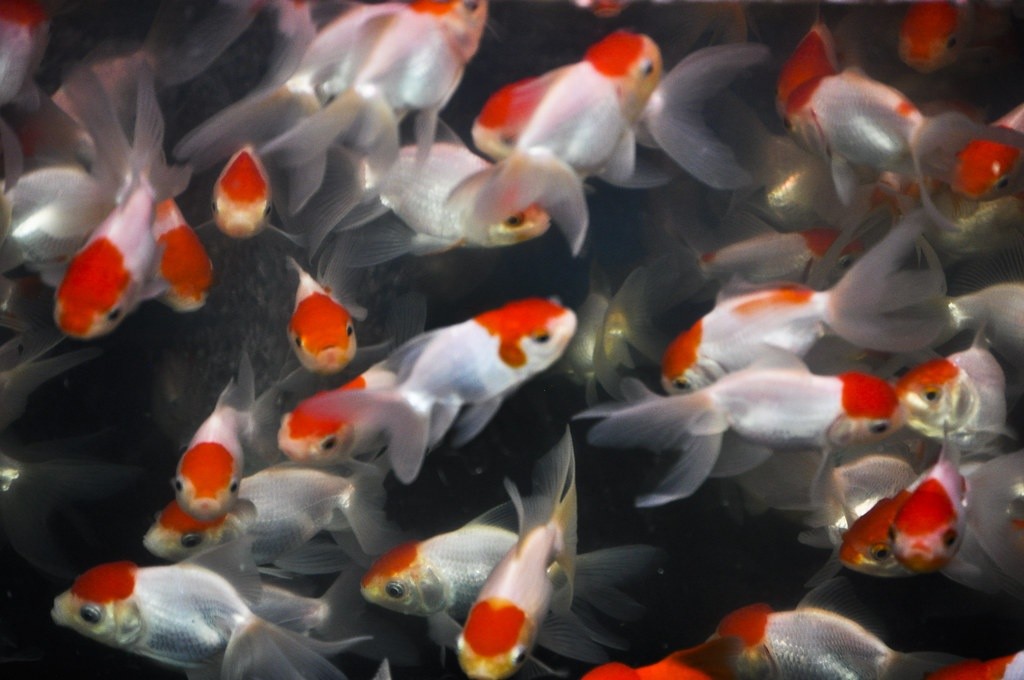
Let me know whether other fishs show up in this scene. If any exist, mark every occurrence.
[0,0,1024,680]
